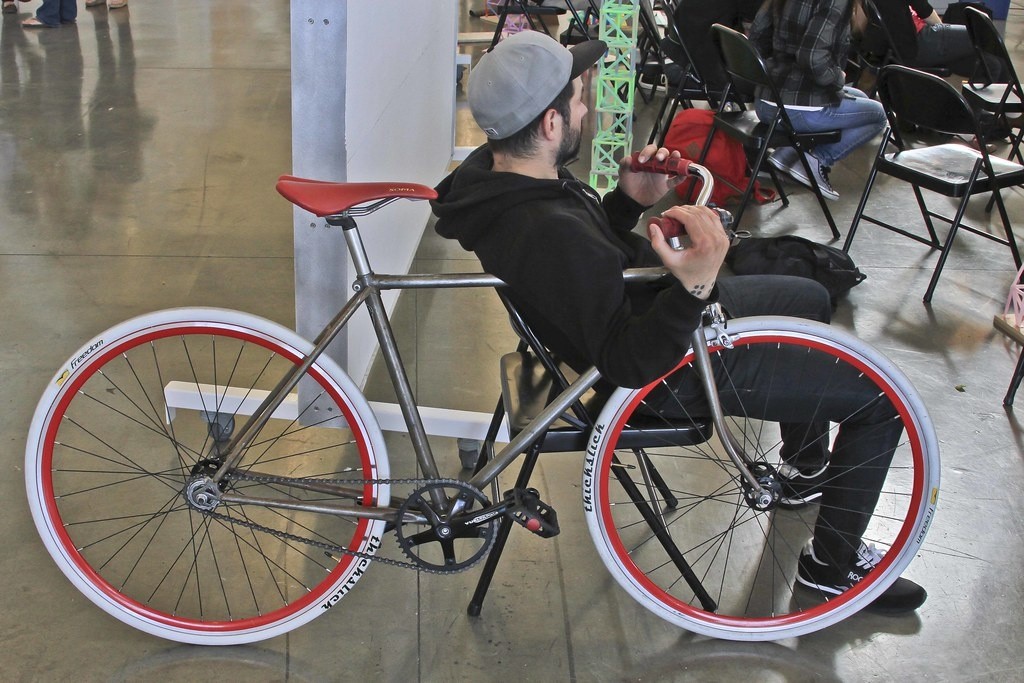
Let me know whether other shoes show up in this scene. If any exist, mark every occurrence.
[22,17,58,28]
[85,0,128,8]
[2,3,18,13]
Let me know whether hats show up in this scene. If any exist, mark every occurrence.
[466,29,608,141]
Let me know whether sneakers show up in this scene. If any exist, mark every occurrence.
[767,145,800,175]
[790,151,841,201]
[773,461,831,510]
[795,537,928,614]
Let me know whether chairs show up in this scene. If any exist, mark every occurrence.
[961,5,1024,214]
[615,1,696,122]
[837,65,1024,305]
[848,0,955,101]
[680,23,845,244]
[558,0,600,49]
[645,0,754,153]
[458,256,718,620]
[487,0,566,54]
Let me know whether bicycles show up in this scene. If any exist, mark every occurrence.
[19,151,943,651]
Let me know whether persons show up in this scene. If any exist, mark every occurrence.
[85,0,128,8]
[871,0,1013,140]
[22,0,77,27]
[428,27,928,617]
[666,0,764,98]
[2,0,32,14]
[746,0,888,202]
